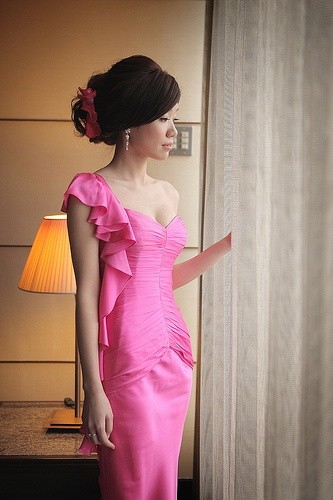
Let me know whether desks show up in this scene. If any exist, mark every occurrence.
[0,403,99,463]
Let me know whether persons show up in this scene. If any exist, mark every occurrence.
[61,53,233,500]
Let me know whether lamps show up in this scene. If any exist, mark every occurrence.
[18,215,84,431]
